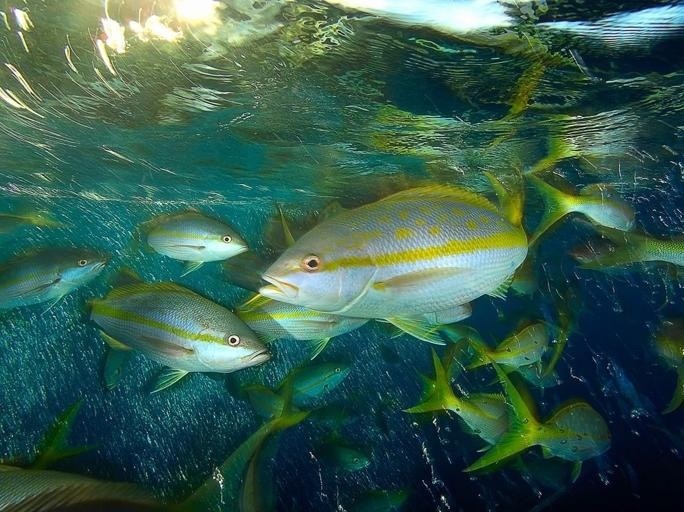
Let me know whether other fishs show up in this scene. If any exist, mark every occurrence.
[1,165,684,512]
